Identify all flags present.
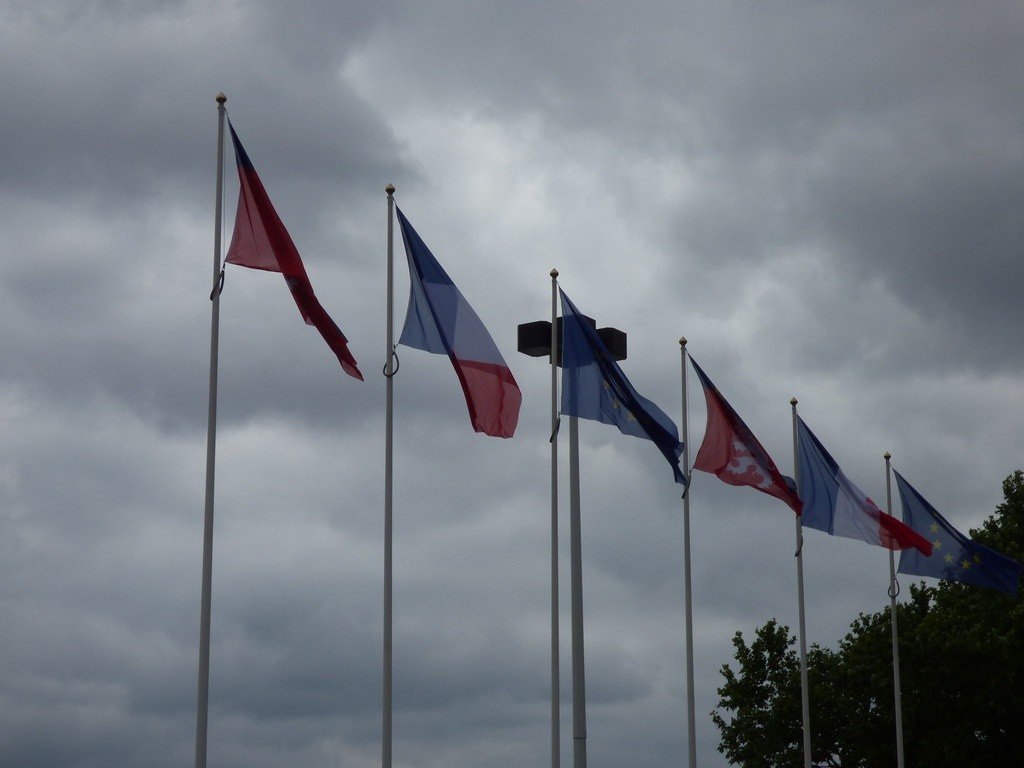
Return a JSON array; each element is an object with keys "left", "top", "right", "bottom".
[
  {"left": 688, "top": 354, "right": 805, "bottom": 517},
  {"left": 225, "top": 118, "right": 364, "bottom": 380},
  {"left": 893, "top": 469, "right": 1024, "bottom": 596},
  {"left": 559, "top": 287, "right": 688, "bottom": 487},
  {"left": 396, "top": 205, "right": 522, "bottom": 438},
  {"left": 797, "top": 415, "right": 933, "bottom": 557}
]
